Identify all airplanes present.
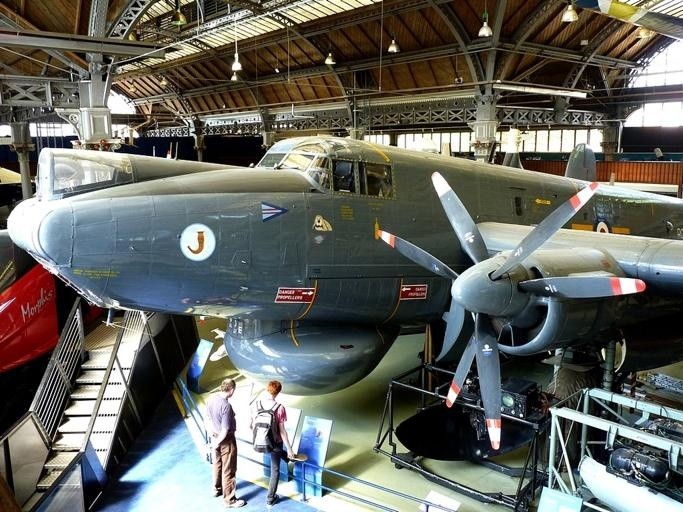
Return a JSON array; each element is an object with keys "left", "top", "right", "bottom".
[{"left": 7, "top": 136, "right": 683, "bottom": 450}]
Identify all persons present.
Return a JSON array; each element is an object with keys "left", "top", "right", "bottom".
[
  {"left": 249, "top": 381, "right": 295, "bottom": 505},
  {"left": 207, "top": 378, "right": 245, "bottom": 508}
]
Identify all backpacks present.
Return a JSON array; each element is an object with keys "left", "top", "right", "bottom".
[{"left": 251, "top": 400, "right": 281, "bottom": 453}]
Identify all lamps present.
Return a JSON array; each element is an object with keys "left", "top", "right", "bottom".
[
  {"left": 478, "top": 0, "right": 492, "bottom": 37},
  {"left": 170, "top": 1, "right": 187, "bottom": 26},
  {"left": 229, "top": 21, "right": 243, "bottom": 81},
  {"left": 636, "top": 27, "right": 652, "bottom": 39},
  {"left": 324, "top": 32, "right": 336, "bottom": 65},
  {"left": 560, "top": 0, "right": 579, "bottom": 23},
  {"left": 387, "top": 16, "right": 399, "bottom": 53}
]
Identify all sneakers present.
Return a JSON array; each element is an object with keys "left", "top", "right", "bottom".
[
  {"left": 265, "top": 494, "right": 280, "bottom": 508},
  {"left": 213, "top": 490, "right": 222, "bottom": 497},
  {"left": 225, "top": 500, "right": 246, "bottom": 509},
  {"left": 287, "top": 454, "right": 308, "bottom": 462}
]
